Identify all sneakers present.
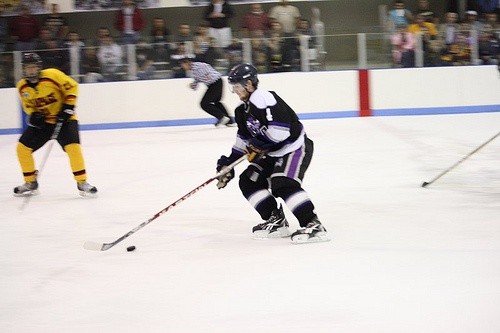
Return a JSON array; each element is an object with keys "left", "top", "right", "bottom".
[
  {"left": 215, "top": 116, "right": 230, "bottom": 128},
  {"left": 292, "top": 214, "right": 331, "bottom": 243},
  {"left": 14, "top": 181, "right": 38, "bottom": 197},
  {"left": 77, "top": 180, "right": 97, "bottom": 197},
  {"left": 226, "top": 119, "right": 238, "bottom": 127},
  {"left": 252, "top": 203, "right": 292, "bottom": 238}
]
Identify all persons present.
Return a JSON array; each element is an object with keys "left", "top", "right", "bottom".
[
  {"left": 181, "top": 56, "right": 235, "bottom": 127},
  {"left": 150, "top": 18, "right": 221, "bottom": 78},
  {"left": 43, "top": 3, "right": 68, "bottom": 43},
  {"left": 12, "top": 52, "right": 99, "bottom": 197},
  {"left": 216, "top": 62, "right": 331, "bottom": 245},
  {"left": 386, "top": 0, "right": 500, "bottom": 67},
  {"left": 202, "top": 0, "right": 325, "bottom": 73},
  {"left": 7, "top": 4, "right": 40, "bottom": 51},
  {"left": 81, "top": 27, "right": 158, "bottom": 83},
  {"left": 112, "top": 0, "right": 145, "bottom": 42},
  {"left": 34, "top": 29, "right": 86, "bottom": 75}
]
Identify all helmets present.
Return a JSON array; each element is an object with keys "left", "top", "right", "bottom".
[
  {"left": 19, "top": 53, "right": 43, "bottom": 80},
  {"left": 179, "top": 57, "right": 193, "bottom": 63},
  {"left": 228, "top": 63, "right": 258, "bottom": 83}
]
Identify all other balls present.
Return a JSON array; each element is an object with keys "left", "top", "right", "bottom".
[{"left": 126, "top": 245, "right": 136, "bottom": 251}]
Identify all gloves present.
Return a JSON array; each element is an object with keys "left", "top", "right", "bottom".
[
  {"left": 56, "top": 104, "right": 74, "bottom": 122},
  {"left": 246, "top": 134, "right": 276, "bottom": 163},
  {"left": 217, "top": 156, "right": 234, "bottom": 189},
  {"left": 30, "top": 112, "right": 46, "bottom": 126}
]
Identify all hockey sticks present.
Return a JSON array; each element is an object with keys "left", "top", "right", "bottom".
[
  {"left": 421, "top": 132, "right": 500, "bottom": 188},
  {"left": 84, "top": 154, "right": 249, "bottom": 251},
  {"left": 15, "top": 117, "right": 64, "bottom": 213}
]
